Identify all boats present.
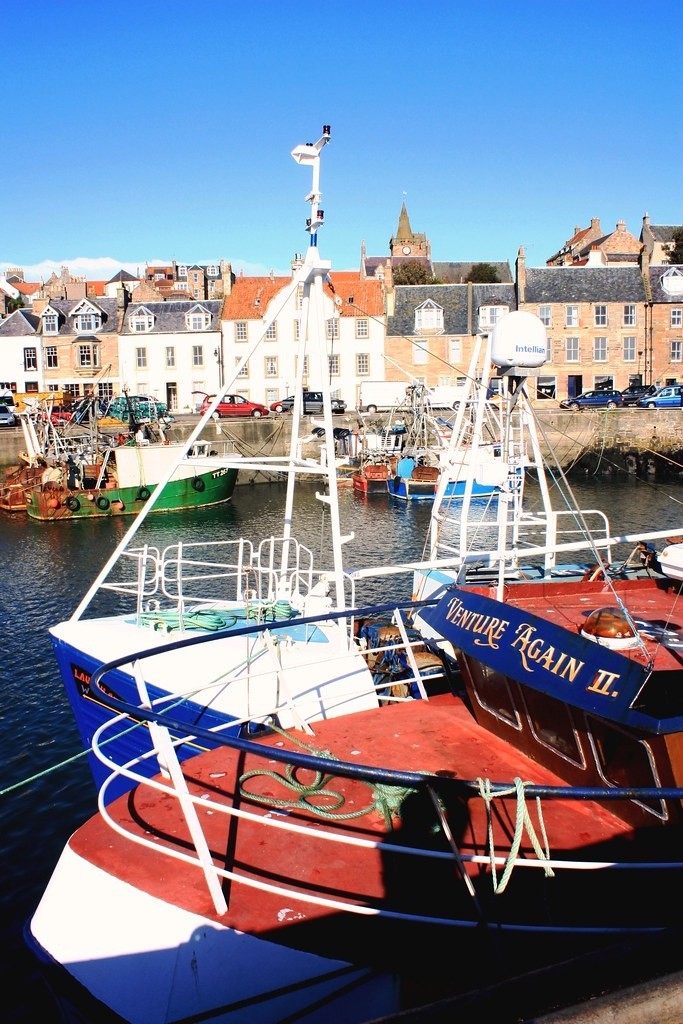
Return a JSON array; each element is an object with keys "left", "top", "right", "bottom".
[
  {"left": 20, "top": 126, "right": 683, "bottom": 1024},
  {"left": 352, "top": 352, "right": 529, "bottom": 500},
  {"left": 0, "top": 363, "right": 243, "bottom": 521}
]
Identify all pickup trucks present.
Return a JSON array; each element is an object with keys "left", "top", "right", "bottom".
[{"left": 282, "top": 391, "right": 347, "bottom": 415}]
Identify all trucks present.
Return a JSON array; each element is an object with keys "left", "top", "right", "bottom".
[{"left": 359, "top": 381, "right": 471, "bottom": 414}]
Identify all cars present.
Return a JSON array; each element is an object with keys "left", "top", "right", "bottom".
[
  {"left": 559, "top": 390, "right": 624, "bottom": 411},
  {"left": 191, "top": 391, "right": 270, "bottom": 419},
  {"left": 0, "top": 404, "right": 15, "bottom": 427},
  {"left": 621, "top": 385, "right": 683, "bottom": 407},
  {"left": 270, "top": 395, "right": 296, "bottom": 413},
  {"left": 42, "top": 406, "right": 72, "bottom": 426}
]
